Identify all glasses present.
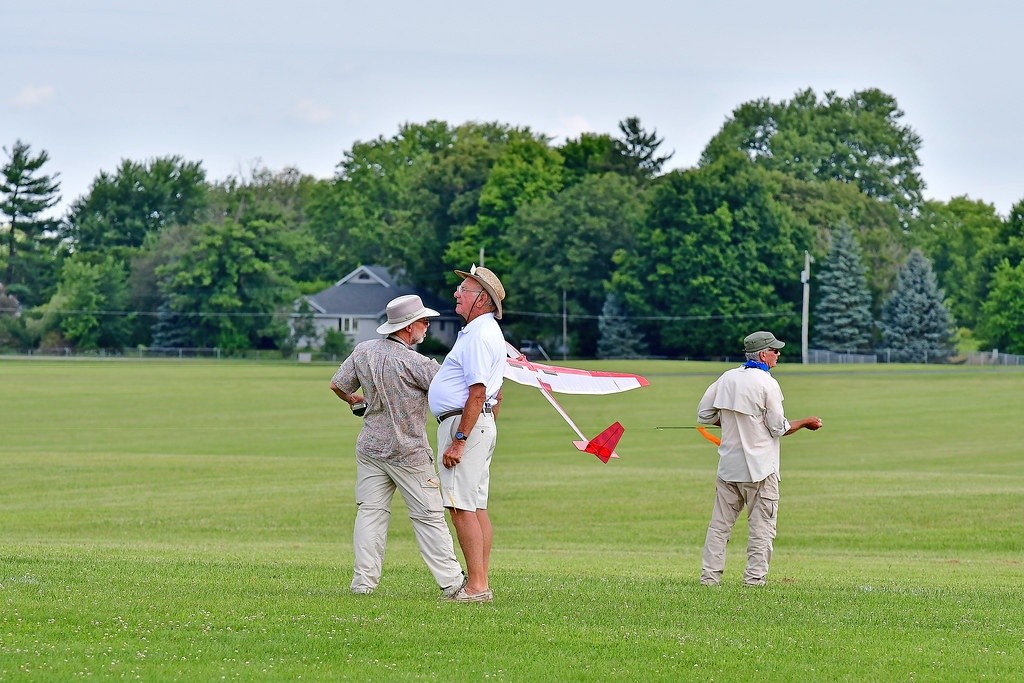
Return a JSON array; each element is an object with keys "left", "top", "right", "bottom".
[
  {"left": 769, "top": 348, "right": 779, "bottom": 354},
  {"left": 457, "top": 285, "right": 488, "bottom": 296},
  {"left": 415, "top": 318, "right": 429, "bottom": 326}
]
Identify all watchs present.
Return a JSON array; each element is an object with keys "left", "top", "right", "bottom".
[{"left": 455, "top": 431, "right": 467, "bottom": 441}]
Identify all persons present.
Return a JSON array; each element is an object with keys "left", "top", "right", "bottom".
[
  {"left": 697, "top": 331, "right": 822, "bottom": 586},
  {"left": 330, "top": 295, "right": 469, "bottom": 597},
  {"left": 428, "top": 267, "right": 506, "bottom": 601}
]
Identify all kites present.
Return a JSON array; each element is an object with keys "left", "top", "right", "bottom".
[{"left": 500, "top": 340, "right": 649, "bottom": 464}]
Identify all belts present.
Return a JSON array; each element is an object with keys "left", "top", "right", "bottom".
[{"left": 437, "top": 407, "right": 491, "bottom": 424}]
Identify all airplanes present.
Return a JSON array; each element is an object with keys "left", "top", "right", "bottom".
[{"left": 502, "top": 341, "right": 650, "bottom": 465}]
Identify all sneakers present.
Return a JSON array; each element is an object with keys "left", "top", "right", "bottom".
[{"left": 444, "top": 588, "right": 494, "bottom": 602}]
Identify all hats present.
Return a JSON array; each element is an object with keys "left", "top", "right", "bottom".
[
  {"left": 744, "top": 332, "right": 785, "bottom": 353},
  {"left": 454, "top": 263, "right": 505, "bottom": 320},
  {"left": 376, "top": 295, "right": 441, "bottom": 335}
]
